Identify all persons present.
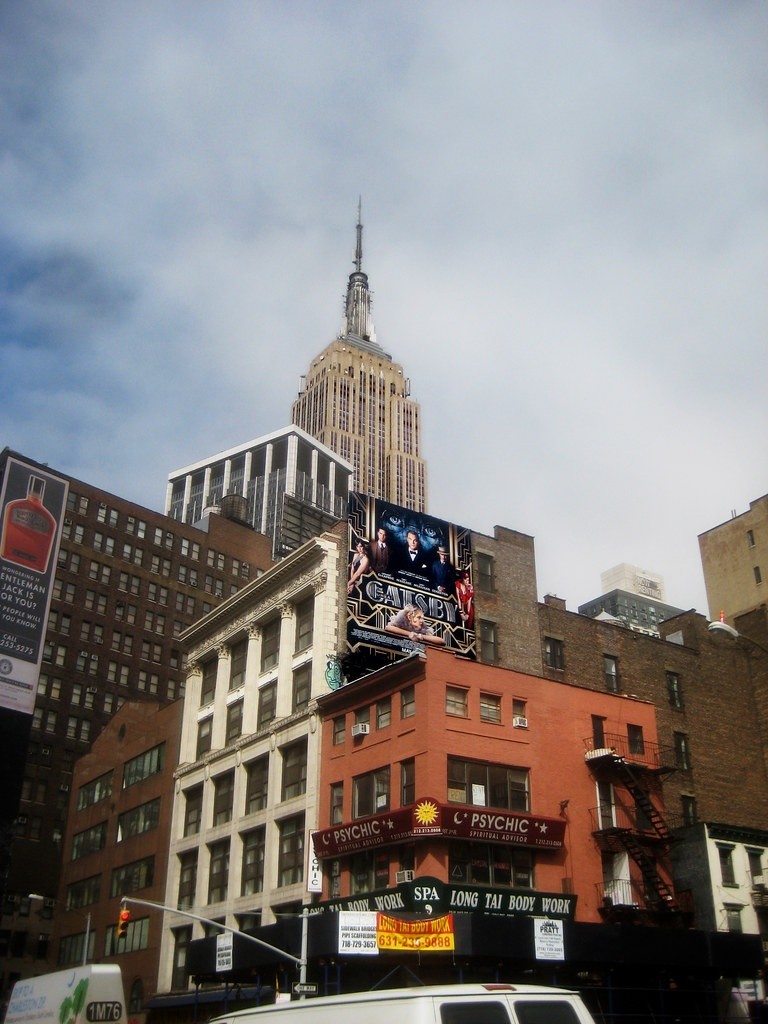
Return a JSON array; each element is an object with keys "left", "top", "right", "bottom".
[
  {"left": 454, "top": 573, "right": 474, "bottom": 629},
  {"left": 430, "top": 545, "right": 456, "bottom": 597},
  {"left": 347, "top": 538, "right": 370, "bottom": 598},
  {"left": 368, "top": 526, "right": 390, "bottom": 575},
  {"left": 385, "top": 602, "right": 446, "bottom": 644},
  {"left": 390, "top": 530, "right": 447, "bottom": 592}
]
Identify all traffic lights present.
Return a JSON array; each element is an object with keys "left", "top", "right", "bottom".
[{"left": 117, "top": 909, "right": 129, "bottom": 938}]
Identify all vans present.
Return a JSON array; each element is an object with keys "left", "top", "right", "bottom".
[{"left": 211, "top": 984, "right": 596, "bottom": 1024}]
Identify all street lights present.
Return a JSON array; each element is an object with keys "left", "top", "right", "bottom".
[{"left": 30, "top": 894, "right": 92, "bottom": 967}]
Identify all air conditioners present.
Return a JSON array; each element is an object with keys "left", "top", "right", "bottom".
[
  {"left": 395, "top": 871, "right": 413, "bottom": 883},
  {"left": 513, "top": 717, "right": 527, "bottom": 728},
  {"left": 351, "top": 724, "right": 369, "bottom": 736},
  {"left": 10, "top": 500, "right": 250, "bottom": 909}
]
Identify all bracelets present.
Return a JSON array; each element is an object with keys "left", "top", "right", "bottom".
[
  {"left": 459, "top": 608, "right": 463, "bottom": 615},
  {"left": 409, "top": 632, "right": 417, "bottom": 639},
  {"left": 418, "top": 633, "right": 424, "bottom": 640}
]
